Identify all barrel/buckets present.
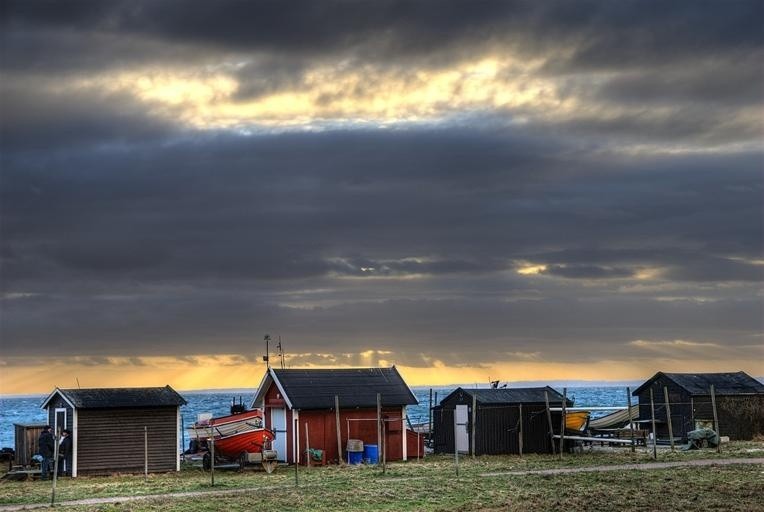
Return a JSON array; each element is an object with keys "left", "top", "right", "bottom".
[
  {"left": 364, "top": 443, "right": 380, "bottom": 465},
  {"left": 346, "top": 450, "right": 364, "bottom": 465}
]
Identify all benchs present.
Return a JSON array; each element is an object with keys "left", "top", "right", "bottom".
[{"left": 611, "top": 425, "right": 649, "bottom": 447}]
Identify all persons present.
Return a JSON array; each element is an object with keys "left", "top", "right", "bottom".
[
  {"left": 59, "top": 430, "right": 72, "bottom": 464},
  {"left": 38, "top": 425, "right": 54, "bottom": 481}
]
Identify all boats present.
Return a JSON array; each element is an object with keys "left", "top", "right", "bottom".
[
  {"left": 565, "top": 404, "right": 639, "bottom": 431},
  {"left": 188, "top": 409, "right": 275, "bottom": 459}
]
